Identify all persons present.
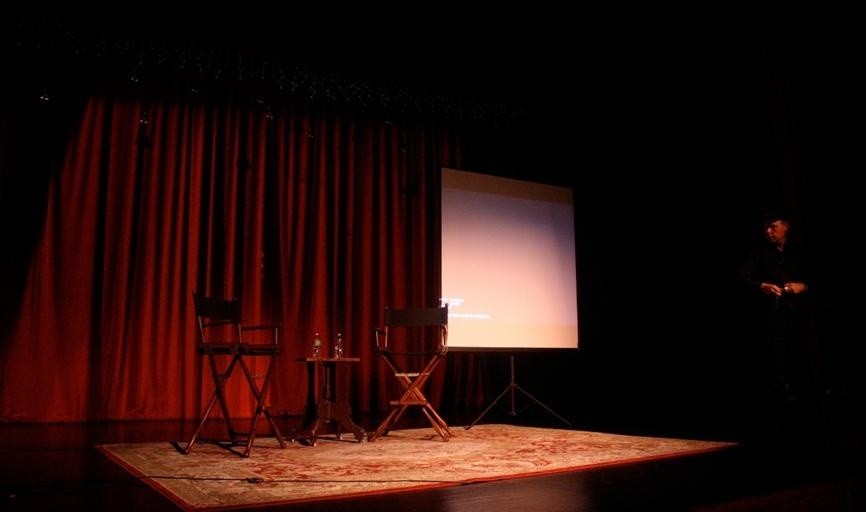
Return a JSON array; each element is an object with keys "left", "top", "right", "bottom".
[{"left": 737, "top": 209, "right": 815, "bottom": 304}]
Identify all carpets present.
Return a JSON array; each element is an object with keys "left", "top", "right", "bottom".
[{"left": 97, "top": 422, "right": 739, "bottom": 512}]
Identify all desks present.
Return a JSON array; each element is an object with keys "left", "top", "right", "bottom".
[{"left": 286, "top": 358, "right": 368, "bottom": 446}]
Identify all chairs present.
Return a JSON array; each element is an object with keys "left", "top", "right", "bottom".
[
  {"left": 185, "top": 289, "right": 287, "bottom": 457},
  {"left": 368, "top": 302, "right": 457, "bottom": 442}
]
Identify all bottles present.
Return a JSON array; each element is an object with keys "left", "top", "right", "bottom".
[
  {"left": 334, "top": 332, "right": 344, "bottom": 360},
  {"left": 311, "top": 332, "right": 323, "bottom": 358}
]
